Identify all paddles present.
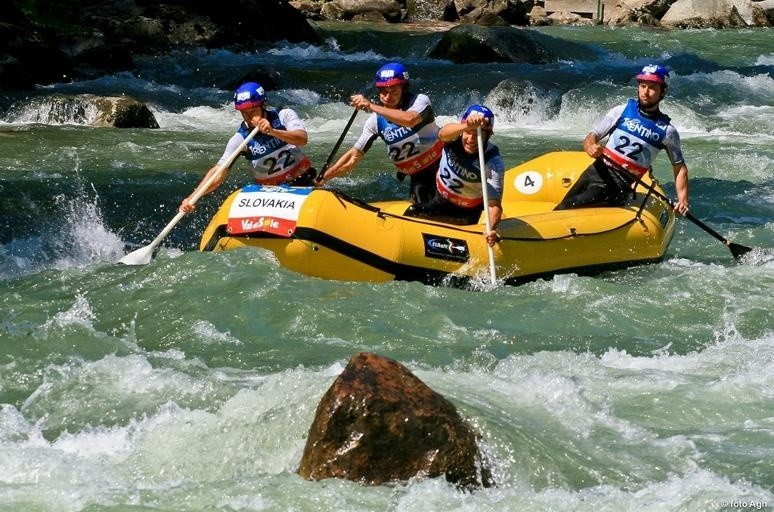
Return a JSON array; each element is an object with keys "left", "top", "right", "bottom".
[
  {"left": 117, "top": 124, "right": 261, "bottom": 266},
  {"left": 599, "top": 155, "right": 753, "bottom": 261}
]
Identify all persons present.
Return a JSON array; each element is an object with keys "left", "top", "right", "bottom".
[
  {"left": 311, "top": 62, "right": 443, "bottom": 203},
  {"left": 552, "top": 64, "right": 691, "bottom": 217},
  {"left": 175, "top": 82, "right": 318, "bottom": 214},
  {"left": 404, "top": 104, "right": 504, "bottom": 245}
]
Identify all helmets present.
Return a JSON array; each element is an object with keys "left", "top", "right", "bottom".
[
  {"left": 461, "top": 105, "right": 495, "bottom": 132},
  {"left": 636, "top": 64, "right": 670, "bottom": 89},
  {"left": 235, "top": 81, "right": 265, "bottom": 110},
  {"left": 374, "top": 63, "right": 410, "bottom": 88}
]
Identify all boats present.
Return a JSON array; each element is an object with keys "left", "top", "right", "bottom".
[{"left": 197, "top": 150, "right": 680, "bottom": 289}]
